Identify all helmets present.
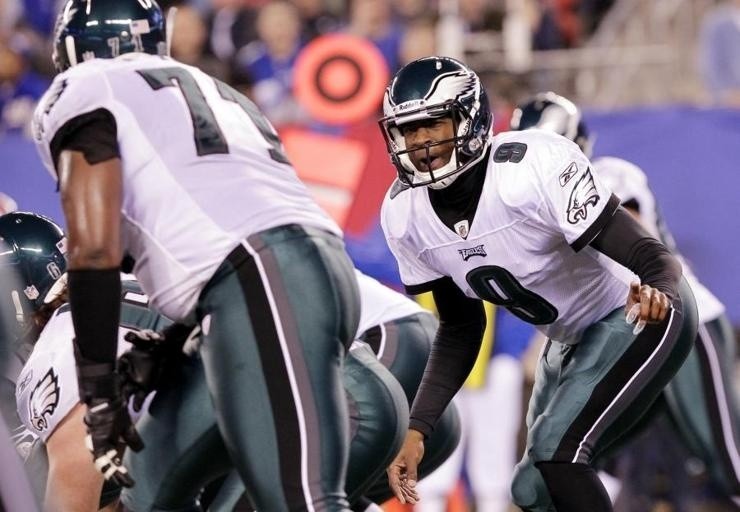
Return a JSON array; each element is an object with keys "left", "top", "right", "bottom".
[
  {"left": 0, "top": 211, "right": 65, "bottom": 323},
  {"left": 51, "top": 1, "right": 166, "bottom": 72},
  {"left": 509, "top": 90, "right": 590, "bottom": 141},
  {"left": 377, "top": 56, "right": 493, "bottom": 174}
]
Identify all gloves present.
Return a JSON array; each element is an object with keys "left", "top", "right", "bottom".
[{"left": 73, "top": 330, "right": 188, "bottom": 489}]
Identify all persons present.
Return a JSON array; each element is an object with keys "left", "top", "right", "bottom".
[
  {"left": 511, "top": 91, "right": 738, "bottom": 512},
  {"left": 29, "top": 1, "right": 359, "bottom": 510},
  {"left": 380, "top": 58, "right": 699, "bottom": 511},
  {"left": 2, "top": 211, "right": 534, "bottom": 511},
  {"left": 1, "top": 2, "right": 617, "bottom": 133}
]
